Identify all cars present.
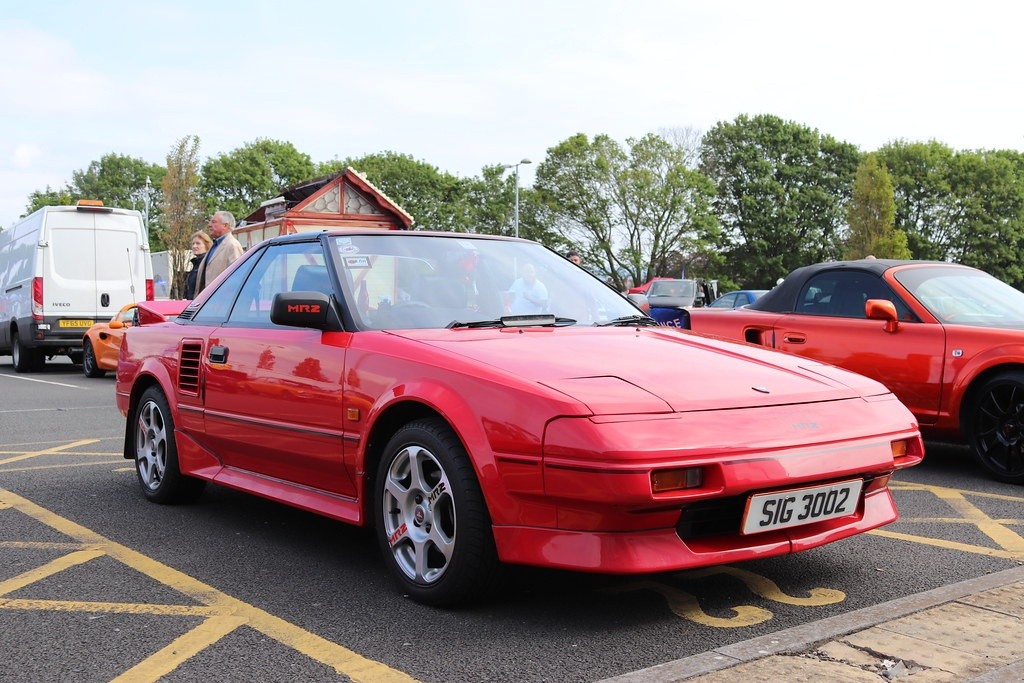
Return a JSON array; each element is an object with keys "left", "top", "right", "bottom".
[
  {"left": 708, "top": 289, "right": 819, "bottom": 309},
  {"left": 646, "top": 278, "right": 713, "bottom": 312}
]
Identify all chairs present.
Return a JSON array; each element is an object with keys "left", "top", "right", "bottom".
[
  {"left": 289, "top": 264, "right": 336, "bottom": 301},
  {"left": 829, "top": 286, "right": 854, "bottom": 316}
]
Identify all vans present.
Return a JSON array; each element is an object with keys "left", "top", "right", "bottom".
[
  {"left": 1, "top": 200, "right": 155, "bottom": 373},
  {"left": 150, "top": 251, "right": 195, "bottom": 299}
]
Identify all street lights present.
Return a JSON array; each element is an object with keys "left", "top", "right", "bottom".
[{"left": 502, "top": 158, "right": 533, "bottom": 276}]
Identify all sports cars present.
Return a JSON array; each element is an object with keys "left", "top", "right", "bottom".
[
  {"left": 680, "top": 259, "right": 1024, "bottom": 487},
  {"left": 113, "top": 222, "right": 925, "bottom": 604},
  {"left": 81, "top": 305, "right": 142, "bottom": 379}
]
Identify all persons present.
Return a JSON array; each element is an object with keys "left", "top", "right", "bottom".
[
  {"left": 194, "top": 211, "right": 244, "bottom": 301},
  {"left": 184, "top": 230, "right": 214, "bottom": 301},
  {"left": 566, "top": 252, "right": 581, "bottom": 267},
  {"left": 504, "top": 263, "right": 549, "bottom": 315}
]
